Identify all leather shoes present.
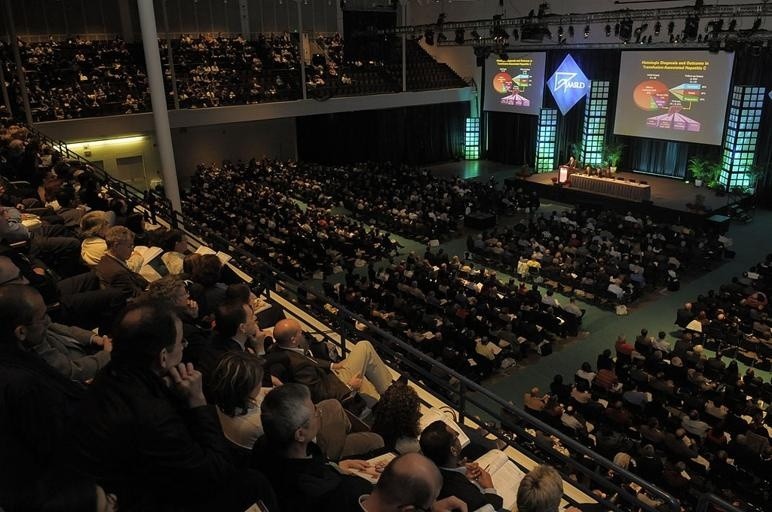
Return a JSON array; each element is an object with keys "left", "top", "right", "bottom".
[{"left": 393, "top": 371, "right": 411, "bottom": 388}]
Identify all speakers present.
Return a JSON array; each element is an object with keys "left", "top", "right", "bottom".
[
  {"left": 706, "top": 214, "right": 730, "bottom": 233},
  {"left": 504, "top": 176, "right": 515, "bottom": 186}
]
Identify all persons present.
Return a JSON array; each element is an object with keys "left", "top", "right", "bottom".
[{"left": 0, "top": 32, "right": 772, "bottom": 512}]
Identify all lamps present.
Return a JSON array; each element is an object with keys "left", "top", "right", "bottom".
[{"left": 408, "top": 1, "right": 772, "bottom": 58}]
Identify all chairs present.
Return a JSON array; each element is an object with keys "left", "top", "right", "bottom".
[
  {"left": 0, "top": 31, "right": 467, "bottom": 124},
  {"left": 0, "top": 126, "right": 772, "bottom": 512}
]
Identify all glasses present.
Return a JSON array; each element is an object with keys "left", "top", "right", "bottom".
[
  {"left": 126, "top": 244, "right": 136, "bottom": 250},
  {"left": 298, "top": 404, "right": 322, "bottom": 428},
  {"left": 449, "top": 431, "right": 461, "bottom": 446},
  {"left": 169, "top": 338, "right": 189, "bottom": 354}
]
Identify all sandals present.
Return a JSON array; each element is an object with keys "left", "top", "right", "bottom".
[
  {"left": 477, "top": 420, "right": 496, "bottom": 438},
  {"left": 496, "top": 437, "right": 509, "bottom": 451}
]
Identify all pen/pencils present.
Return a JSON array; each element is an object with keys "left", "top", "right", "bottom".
[{"left": 476, "top": 464, "right": 489, "bottom": 481}]
